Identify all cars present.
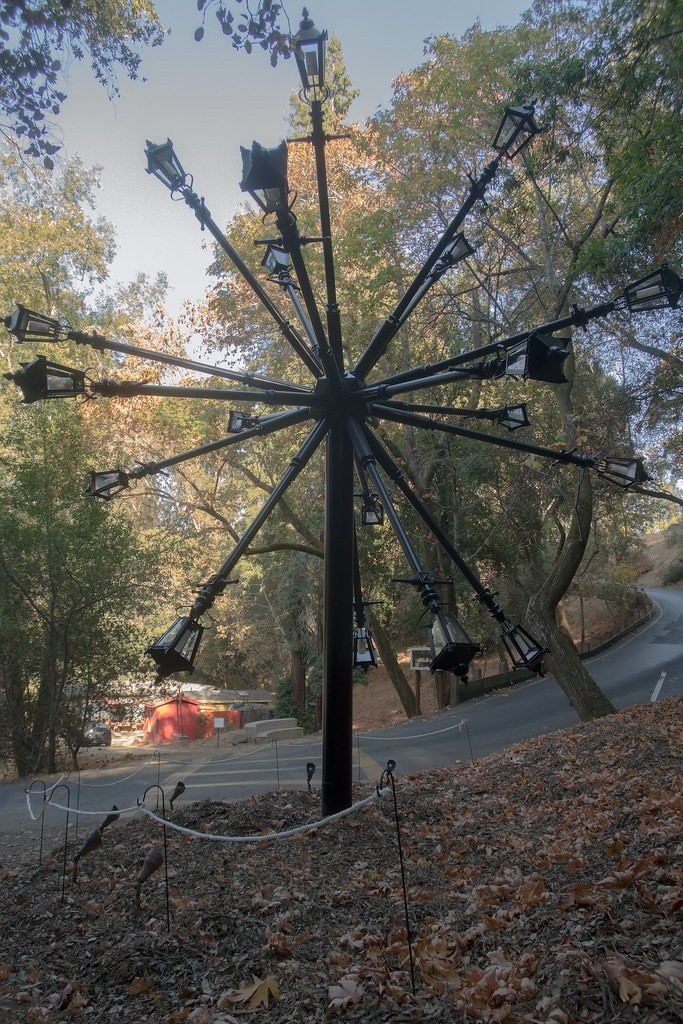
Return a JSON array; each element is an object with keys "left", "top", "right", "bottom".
[{"left": 70, "top": 722, "right": 111, "bottom": 748}]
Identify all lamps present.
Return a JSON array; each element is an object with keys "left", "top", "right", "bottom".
[
  {"left": 73, "top": 828, "right": 101, "bottom": 882},
  {"left": 100, "top": 805, "right": 121, "bottom": 830},
  {"left": 386, "top": 759, "right": 396, "bottom": 784},
  {"left": 306, "top": 763, "right": 316, "bottom": 791},
  {"left": 133, "top": 847, "right": 164, "bottom": 915},
  {"left": 169, "top": 781, "right": 185, "bottom": 810}
]
